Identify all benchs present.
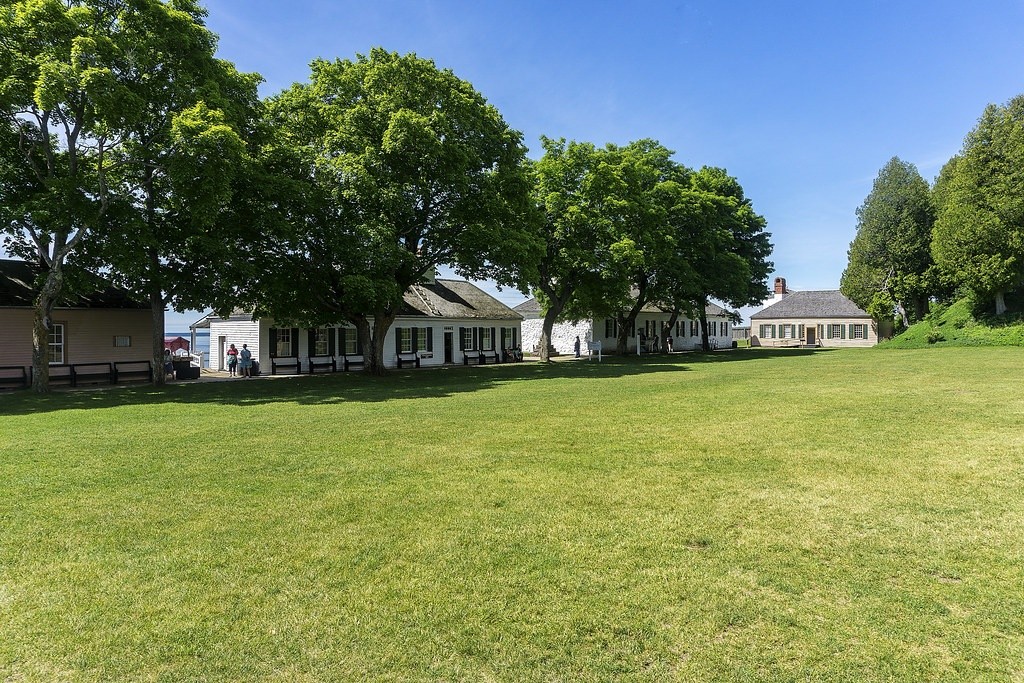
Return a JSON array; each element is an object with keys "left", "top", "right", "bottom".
[
  {"left": 309, "top": 356, "right": 337, "bottom": 374},
  {"left": 272, "top": 356, "right": 301, "bottom": 375},
  {"left": 72, "top": 362, "right": 113, "bottom": 385},
  {"left": 463, "top": 349, "right": 481, "bottom": 365},
  {"left": 30, "top": 364, "right": 75, "bottom": 386},
  {"left": 0, "top": 366, "right": 28, "bottom": 389},
  {"left": 481, "top": 350, "right": 500, "bottom": 364},
  {"left": 396, "top": 352, "right": 421, "bottom": 368},
  {"left": 114, "top": 361, "right": 153, "bottom": 383},
  {"left": 502, "top": 349, "right": 520, "bottom": 363},
  {"left": 344, "top": 355, "right": 365, "bottom": 371}
]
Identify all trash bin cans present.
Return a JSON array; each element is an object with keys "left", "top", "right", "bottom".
[
  {"left": 237, "top": 358, "right": 256, "bottom": 376},
  {"left": 732, "top": 341, "right": 737, "bottom": 348}
]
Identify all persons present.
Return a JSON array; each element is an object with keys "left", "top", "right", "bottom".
[
  {"left": 240, "top": 344, "right": 252, "bottom": 378},
  {"left": 507, "top": 346, "right": 517, "bottom": 363},
  {"left": 668, "top": 336, "right": 673, "bottom": 352},
  {"left": 227, "top": 344, "right": 238, "bottom": 377},
  {"left": 574, "top": 336, "right": 580, "bottom": 358},
  {"left": 164, "top": 350, "right": 174, "bottom": 381},
  {"left": 653, "top": 335, "right": 659, "bottom": 352},
  {"left": 515, "top": 344, "right": 524, "bottom": 362}
]
